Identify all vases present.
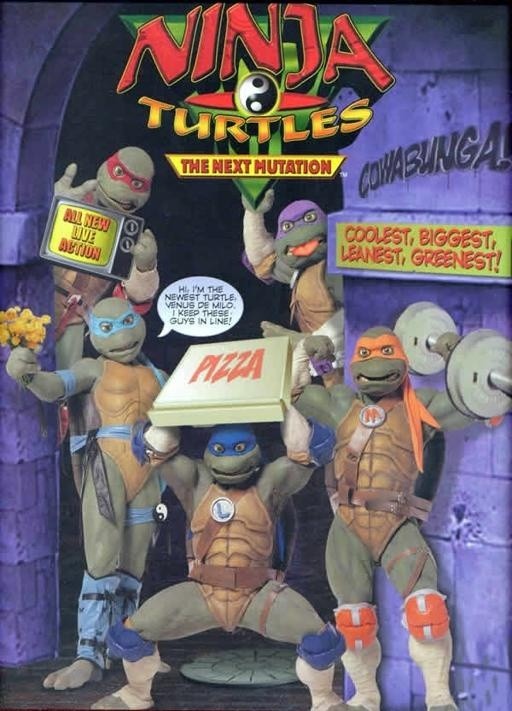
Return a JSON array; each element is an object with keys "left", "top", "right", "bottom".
[{"left": 0, "top": 304, "right": 52, "bottom": 389}]
